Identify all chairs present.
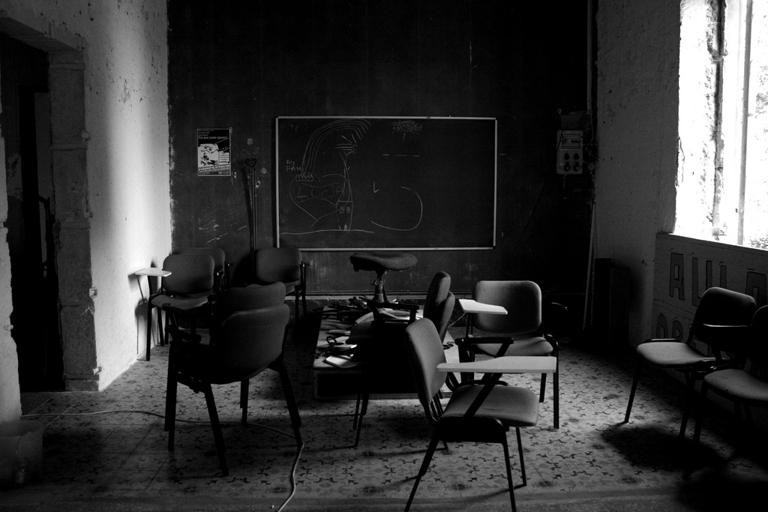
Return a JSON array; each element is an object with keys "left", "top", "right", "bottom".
[
  {"left": 624, "top": 287, "right": 757, "bottom": 438},
  {"left": 162, "top": 283, "right": 286, "bottom": 431},
  {"left": 679, "top": 305, "right": 766, "bottom": 481},
  {"left": 168, "top": 305, "right": 304, "bottom": 477},
  {"left": 472, "top": 279, "right": 561, "bottom": 433},
  {"left": 179, "top": 250, "right": 232, "bottom": 293},
  {"left": 351, "top": 272, "right": 458, "bottom": 455},
  {"left": 146, "top": 253, "right": 215, "bottom": 361},
  {"left": 255, "top": 248, "right": 308, "bottom": 324},
  {"left": 404, "top": 317, "right": 537, "bottom": 511}
]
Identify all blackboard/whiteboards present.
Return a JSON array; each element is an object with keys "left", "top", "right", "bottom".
[{"left": 276, "top": 116, "right": 497, "bottom": 251}]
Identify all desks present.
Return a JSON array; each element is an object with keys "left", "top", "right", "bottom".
[{"left": 350, "top": 252, "right": 418, "bottom": 303}]
